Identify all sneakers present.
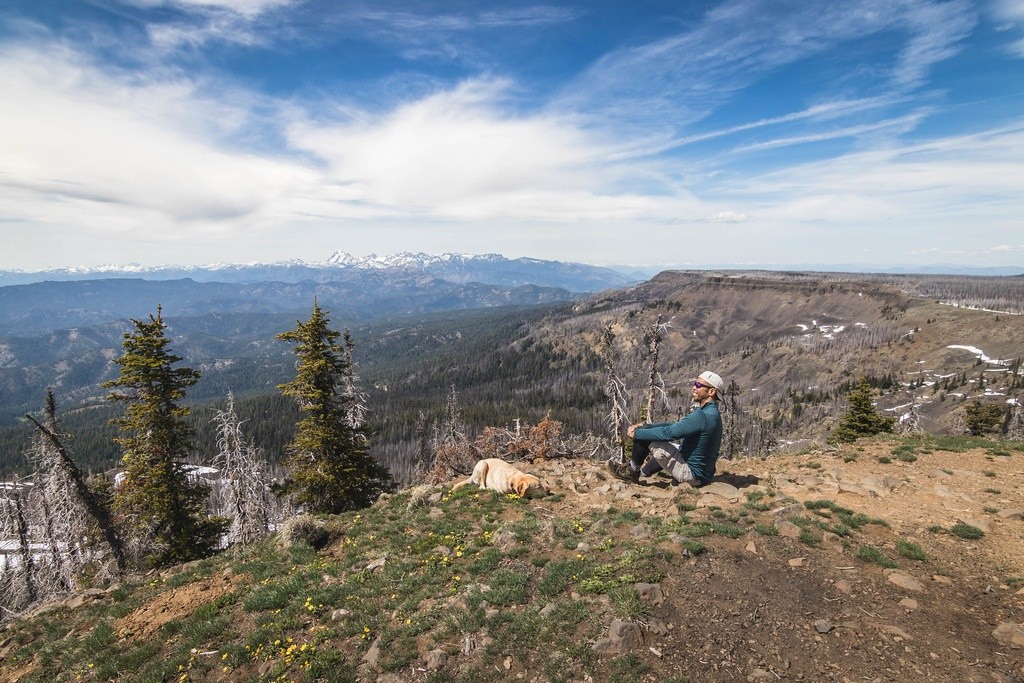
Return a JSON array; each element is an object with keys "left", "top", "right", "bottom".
[
  {"left": 640, "top": 455, "right": 650, "bottom": 477},
  {"left": 605, "top": 460, "right": 640, "bottom": 484}
]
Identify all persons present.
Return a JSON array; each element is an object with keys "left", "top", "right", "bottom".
[{"left": 606, "top": 371, "right": 725, "bottom": 487}]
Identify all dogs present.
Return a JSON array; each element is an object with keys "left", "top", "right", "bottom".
[{"left": 451, "top": 458, "right": 550, "bottom": 502}]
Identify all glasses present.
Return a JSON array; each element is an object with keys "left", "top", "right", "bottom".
[{"left": 694, "top": 381, "right": 715, "bottom": 389}]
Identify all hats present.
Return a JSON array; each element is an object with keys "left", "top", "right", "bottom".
[{"left": 698, "top": 371, "right": 724, "bottom": 402}]
